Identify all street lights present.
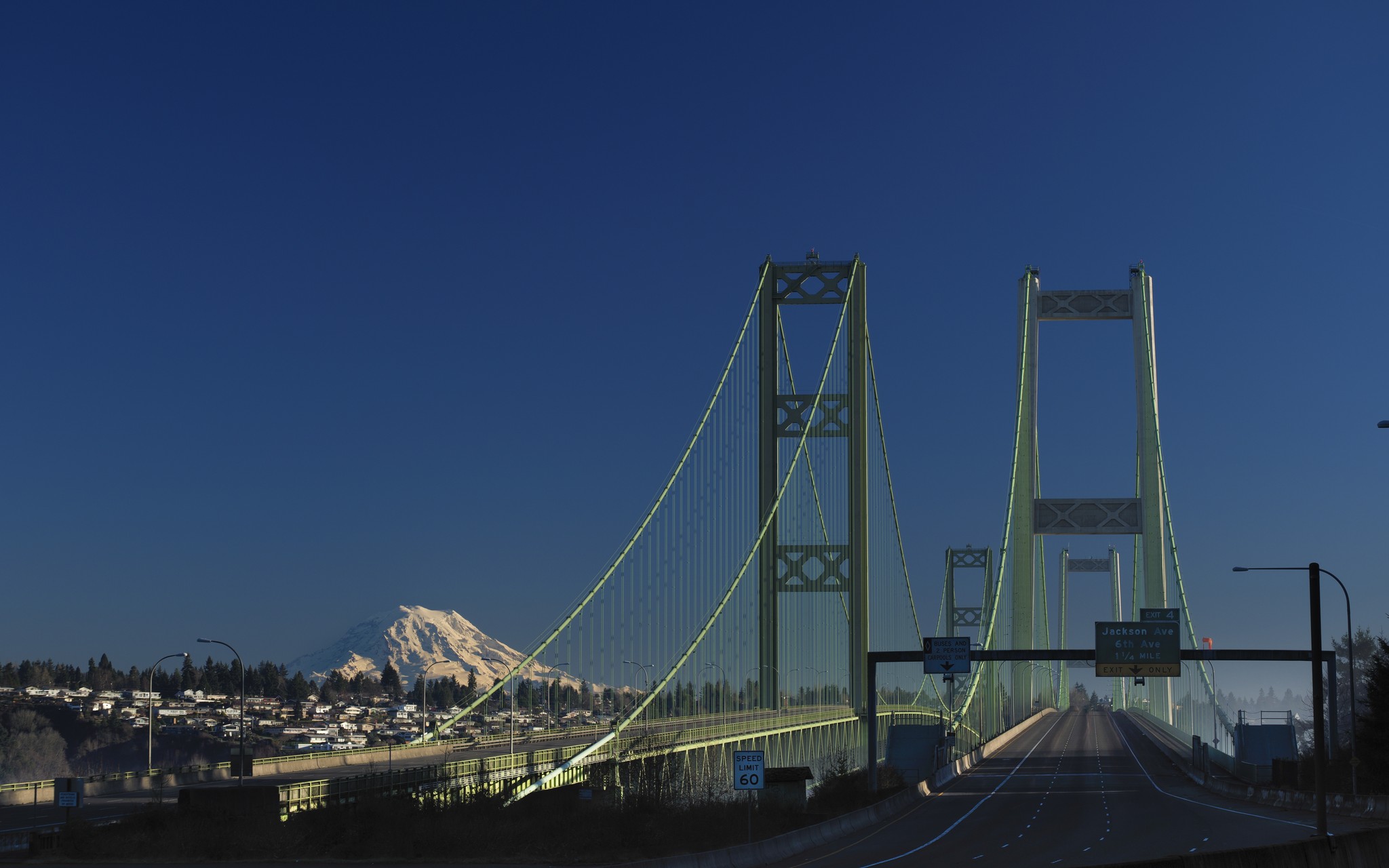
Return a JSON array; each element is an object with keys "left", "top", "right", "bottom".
[
  {"left": 887, "top": 673, "right": 899, "bottom": 705},
  {"left": 905, "top": 676, "right": 916, "bottom": 709},
  {"left": 548, "top": 663, "right": 571, "bottom": 730},
  {"left": 746, "top": 668, "right": 760, "bottom": 710},
  {"left": 623, "top": 661, "right": 654, "bottom": 735},
  {"left": 876, "top": 673, "right": 888, "bottom": 706},
  {"left": 953, "top": 697, "right": 960, "bottom": 709},
  {"left": 149, "top": 653, "right": 188, "bottom": 778},
  {"left": 1105, "top": 680, "right": 1129, "bottom": 711},
  {"left": 838, "top": 668, "right": 851, "bottom": 706},
  {"left": 920, "top": 681, "right": 929, "bottom": 708},
  {"left": 197, "top": 638, "right": 246, "bottom": 786},
  {"left": 481, "top": 657, "right": 513, "bottom": 753},
  {"left": 805, "top": 667, "right": 829, "bottom": 713},
  {"left": 422, "top": 660, "right": 451, "bottom": 741},
  {"left": 763, "top": 666, "right": 780, "bottom": 718},
  {"left": 698, "top": 664, "right": 726, "bottom": 724},
  {"left": 787, "top": 669, "right": 799, "bottom": 711},
  {"left": 1012, "top": 661, "right": 1070, "bottom": 726},
  {"left": 949, "top": 643, "right": 983, "bottom": 762},
  {"left": 934, "top": 686, "right": 941, "bottom": 708},
  {"left": 1233, "top": 566, "right": 1358, "bottom": 794}
]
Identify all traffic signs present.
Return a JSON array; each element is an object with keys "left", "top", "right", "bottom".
[
  {"left": 922, "top": 637, "right": 971, "bottom": 675},
  {"left": 1095, "top": 621, "right": 1182, "bottom": 677}
]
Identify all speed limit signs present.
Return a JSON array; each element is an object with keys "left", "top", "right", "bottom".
[{"left": 734, "top": 750, "right": 766, "bottom": 790}]
